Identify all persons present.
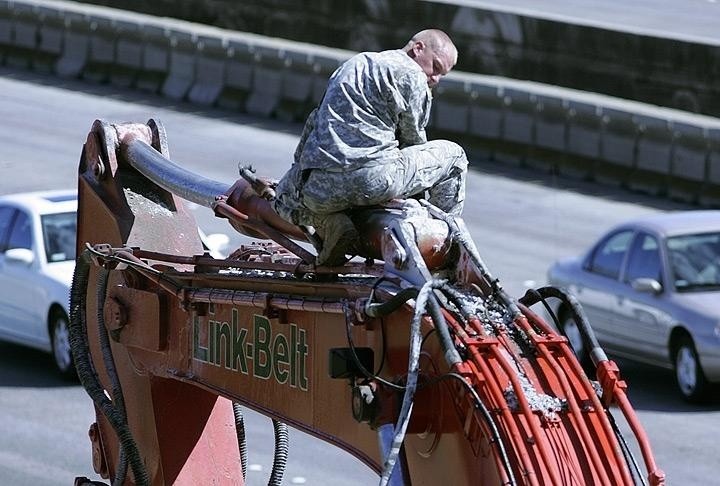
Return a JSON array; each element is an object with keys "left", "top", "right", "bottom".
[{"left": 273, "top": 25, "right": 469, "bottom": 283}]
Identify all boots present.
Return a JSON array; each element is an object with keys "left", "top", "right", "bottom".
[{"left": 310, "top": 211, "right": 359, "bottom": 267}]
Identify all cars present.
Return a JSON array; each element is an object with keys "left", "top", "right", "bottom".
[
  {"left": 542, "top": 208, "right": 719, "bottom": 406},
  {"left": 0, "top": 189, "right": 231, "bottom": 376}
]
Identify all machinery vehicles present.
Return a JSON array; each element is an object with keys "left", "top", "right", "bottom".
[{"left": 70, "top": 118, "right": 665, "bottom": 486}]
